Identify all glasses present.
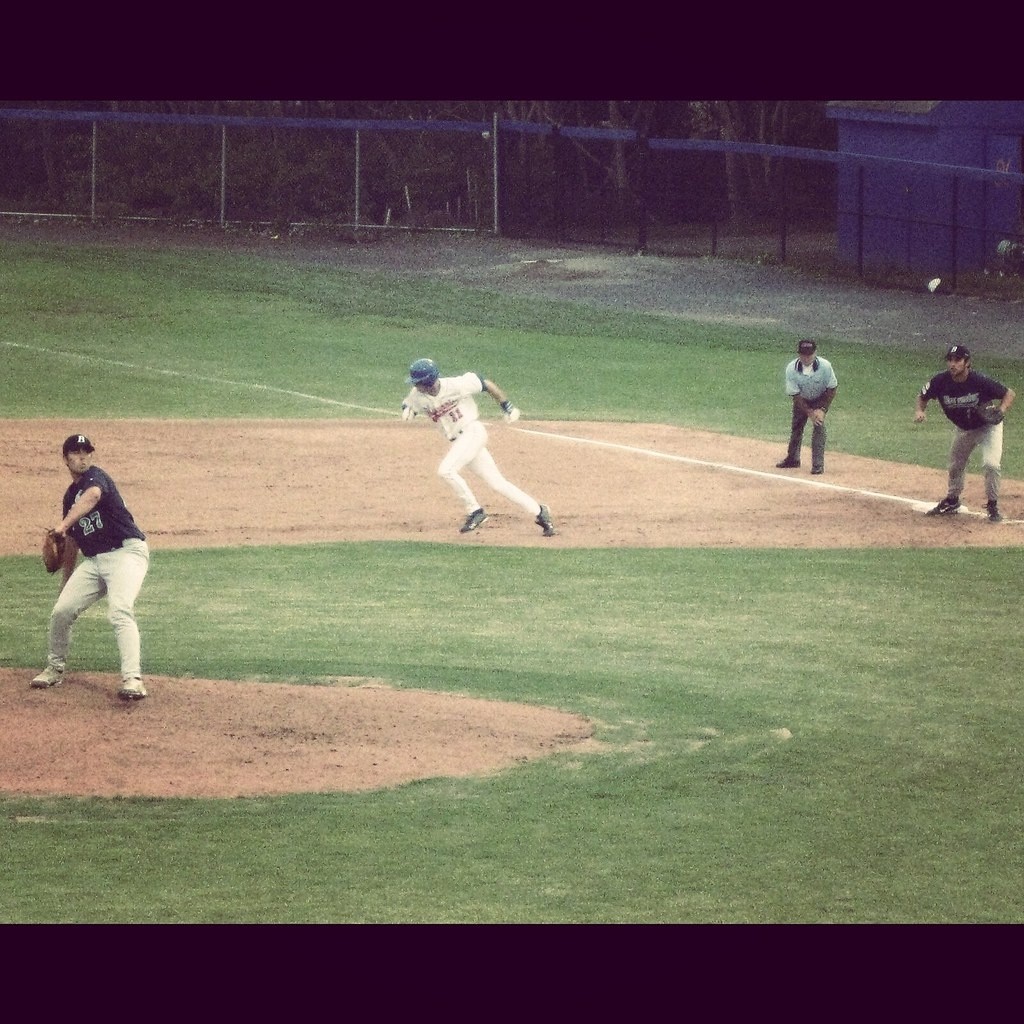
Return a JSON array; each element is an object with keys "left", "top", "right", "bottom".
[{"left": 67, "top": 450, "right": 90, "bottom": 456}]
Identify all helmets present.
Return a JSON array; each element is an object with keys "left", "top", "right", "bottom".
[{"left": 405, "top": 358, "right": 440, "bottom": 387}]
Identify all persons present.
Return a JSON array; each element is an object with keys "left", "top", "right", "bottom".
[
  {"left": 28, "top": 434, "right": 150, "bottom": 701},
  {"left": 914, "top": 347, "right": 1016, "bottom": 522},
  {"left": 776, "top": 340, "right": 839, "bottom": 475},
  {"left": 401, "top": 358, "right": 555, "bottom": 537}
]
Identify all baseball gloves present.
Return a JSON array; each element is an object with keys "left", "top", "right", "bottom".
[
  {"left": 977, "top": 400, "right": 1005, "bottom": 426},
  {"left": 42, "top": 525, "right": 66, "bottom": 577}
]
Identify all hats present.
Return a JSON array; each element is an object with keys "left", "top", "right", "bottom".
[
  {"left": 63, "top": 434, "right": 95, "bottom": 456},
  {"left": 945, "top": 345, "right": 970, "bottom": 359},
  {"left": 797, "top": 340, "right": 817, "bottom": 355}
]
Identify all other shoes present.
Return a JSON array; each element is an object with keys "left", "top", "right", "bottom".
[
  {"left": 31, "top": 666, "right": 65, "bottom": 689},
  {"left": 118, "top": 677, "right": 147, "bottom": 699},
  {"left": 534, "top": 505, "right": 555, "bottom": 537},
  {"left": 776, "top": 456, "right": 800, "bottom": 468},
  {"left": 811, "top": 466, "right": 824, "bottom": 474},
  {"left": 982, "top": 500, "right": 1003, "bottom": 522},
  {"left": 460, "top": 508, "right": 488, "bottom": 533},
  {"left": 926, "top": 496, "right": 962, "bottom": 516}
]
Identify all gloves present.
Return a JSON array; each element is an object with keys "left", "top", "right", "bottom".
[
  {"left": 504, "top": 407, "right": 520, "bottom": 424},
  {"left": 401, "top": 407, "right": 415, "bottom": 422}
]
